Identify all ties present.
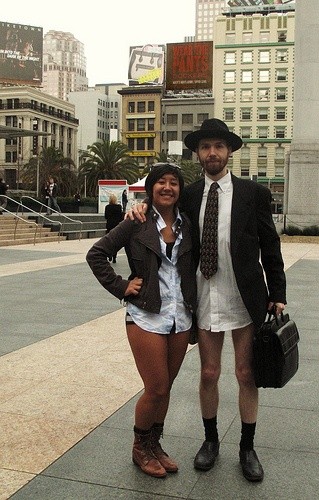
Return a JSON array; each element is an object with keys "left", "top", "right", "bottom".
[{"left": 200, "top": 182, "right": 219, "bottom": 280}]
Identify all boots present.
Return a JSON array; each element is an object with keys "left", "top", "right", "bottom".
[
  {"left": 132, "top": 425, "right": 167, "bottom": 478},
  {"left": 151, "top": 424, "right": 178, "bottom": 473}
]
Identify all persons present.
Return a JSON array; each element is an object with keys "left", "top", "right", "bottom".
[
  {"left": 74, "top": 191, "right": 81, "bottom": 202},
  {"left": 85, "top": 163, "right": 193, "bottom": 478},
  {"left": 122, "top": 190, "right": 128, "bottom": 213},
  {"left": 46, "top": 177, "right": 61, "bottom": 215},
  {"left": 104, "top": 194, "right": 123, "bottom": 264},
  {"left": 124, "top": 117, "right": 286, "bottom": 482},
  {"left": 40, "top": 181, "right": 49, "bottom": 214},
  {"left": 0, "top": 178, "right": 9, "bottom": 214}
]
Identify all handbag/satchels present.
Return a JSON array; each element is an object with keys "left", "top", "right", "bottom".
[{"left": 251, "top": 305, "right": 301, "bottom": 388}]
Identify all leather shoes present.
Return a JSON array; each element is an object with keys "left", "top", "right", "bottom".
[
  {"left": 239, "top": 450, "right": 264, "bottom": 481},
  {"left": 194, "top": 440, "right": 220, "bottom": 469}
]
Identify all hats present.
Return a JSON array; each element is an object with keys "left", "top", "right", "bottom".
[{"left": 185, "top": 119, "right": 243, "bottom": 152}]
